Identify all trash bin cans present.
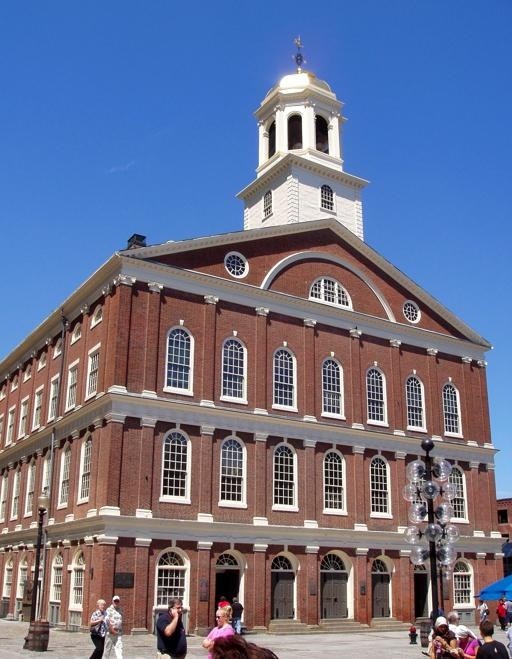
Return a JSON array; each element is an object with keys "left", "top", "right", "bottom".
[
  {"left": 419, "top": 622, "right": 432, "bottom": 647},
  {"left": 28, "top": 621, "right": 49, "bottom": 652}
]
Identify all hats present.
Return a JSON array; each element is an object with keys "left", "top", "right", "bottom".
[
  {"left": 112, "top": 595, "right": 121, "bottom": 602},
  {"left": 435, "top": 615, "right": 449, "bottom": 629}
]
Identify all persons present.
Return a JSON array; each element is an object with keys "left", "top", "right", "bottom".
[
  {"left": 89, "top": 599, "right": 116, "bottom": 659},
  {"left": 101, "top": 595, "right": 123, "bottom": 659},
  {"left": 155, "top": 599, "right": 187, "bottom": 659},
  {"left": 427, "top": 596, "right": 512, "bottom": 659},
  {"left": 202, "top": 596, "right": 280, "bottom": 659}
]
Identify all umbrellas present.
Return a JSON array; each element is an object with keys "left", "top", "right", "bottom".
[{"left": 473, "top": 575, "right": 512, "bottom": 602}]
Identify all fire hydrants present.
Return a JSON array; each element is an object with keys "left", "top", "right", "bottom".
[{"left": 408, "top": 624, "right": 420, "bottom": 645}]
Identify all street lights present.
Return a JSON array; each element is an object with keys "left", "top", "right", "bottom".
[
  {"left": 401, "top": 435, "right": 460, "bottom": 649},
  {"left": 19, "top": 490, "right": 51, "bottom": 649}
]
